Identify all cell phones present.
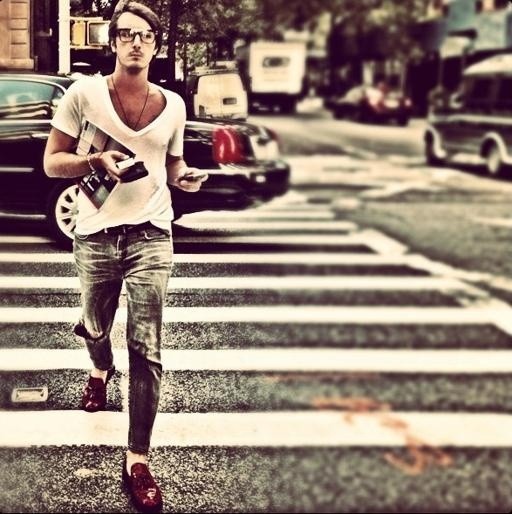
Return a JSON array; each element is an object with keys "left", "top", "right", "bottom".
[{"left": 177, "top": 173, "right": 207, "bottom": 183}]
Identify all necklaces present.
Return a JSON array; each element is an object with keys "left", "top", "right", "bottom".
[{"left": 110, "top": 72, "right": 151, "bottom": 131}]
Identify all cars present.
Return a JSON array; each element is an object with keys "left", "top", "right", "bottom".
[
  {"left": 0, "top": 71, "right": 290, "bottom": 250},
  {"left": 334, "top": 85, "right": 414, "bottom": 125},
  {"left": 424, "top": 53, "right": 512, "bottom": 178}
]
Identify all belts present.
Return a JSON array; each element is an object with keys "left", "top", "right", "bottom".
[{"left": 103, "top": 224, "right": 128, "bottom": 237}]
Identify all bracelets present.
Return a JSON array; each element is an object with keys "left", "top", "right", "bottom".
[{"left": 87, "top": 151, "right": 97, "bottom": 174}]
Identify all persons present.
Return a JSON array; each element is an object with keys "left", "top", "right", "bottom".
[{"left": 43, "top": 0, "right": 211, "bottom": 511}]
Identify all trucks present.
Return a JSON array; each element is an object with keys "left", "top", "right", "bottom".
[{"left": 235, "top": 41, "right": 307, "bottom": 114}]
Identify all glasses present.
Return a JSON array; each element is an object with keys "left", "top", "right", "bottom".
[{"left": 116, "top": 28, "right": 158, "bottom": 44}]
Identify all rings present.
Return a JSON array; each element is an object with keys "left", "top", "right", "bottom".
[{"left": 110, "top": 172, "right": 113, "bottom": 178}]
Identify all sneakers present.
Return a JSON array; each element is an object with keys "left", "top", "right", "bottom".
[
  {"left": 122, "top": 454, "right": 162, "bottom": 513},
  {"left": 82, "top": 366, "right": 115, "bottom": 412}
]
